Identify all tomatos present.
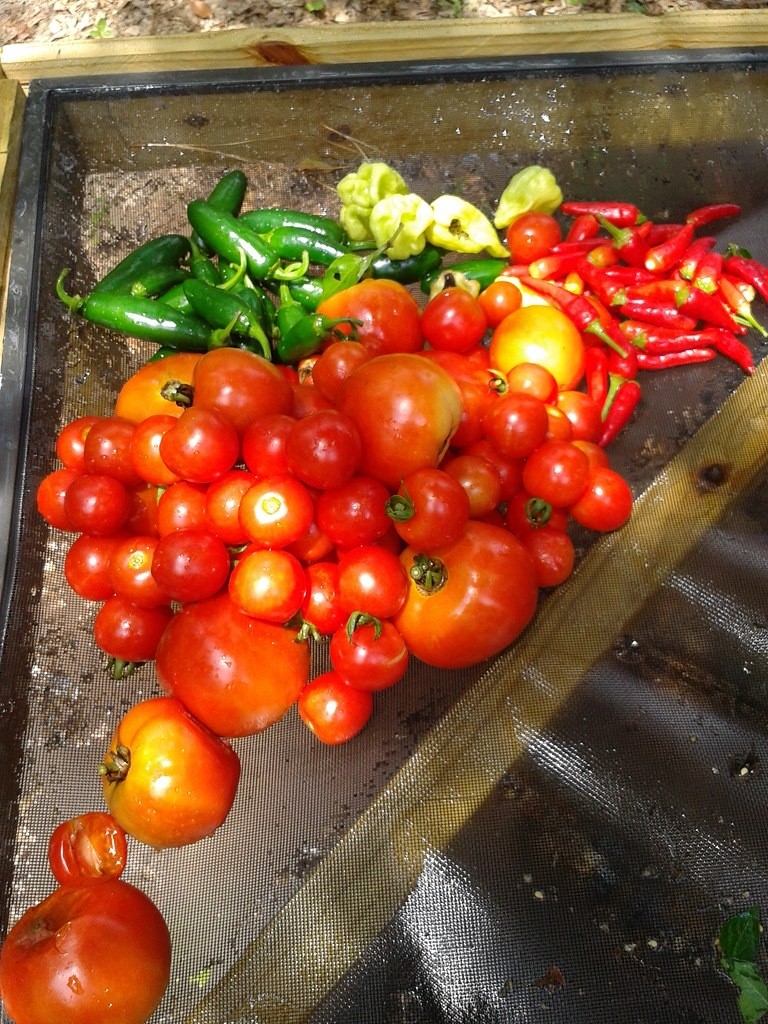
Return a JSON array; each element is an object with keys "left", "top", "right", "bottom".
[{"left": 0, "top": 282, "right": 634, "bottom": 1024}]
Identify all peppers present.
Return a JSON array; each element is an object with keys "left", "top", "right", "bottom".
[{"left": 58, "top": 162, "right": 768, "bottom": 450}]
